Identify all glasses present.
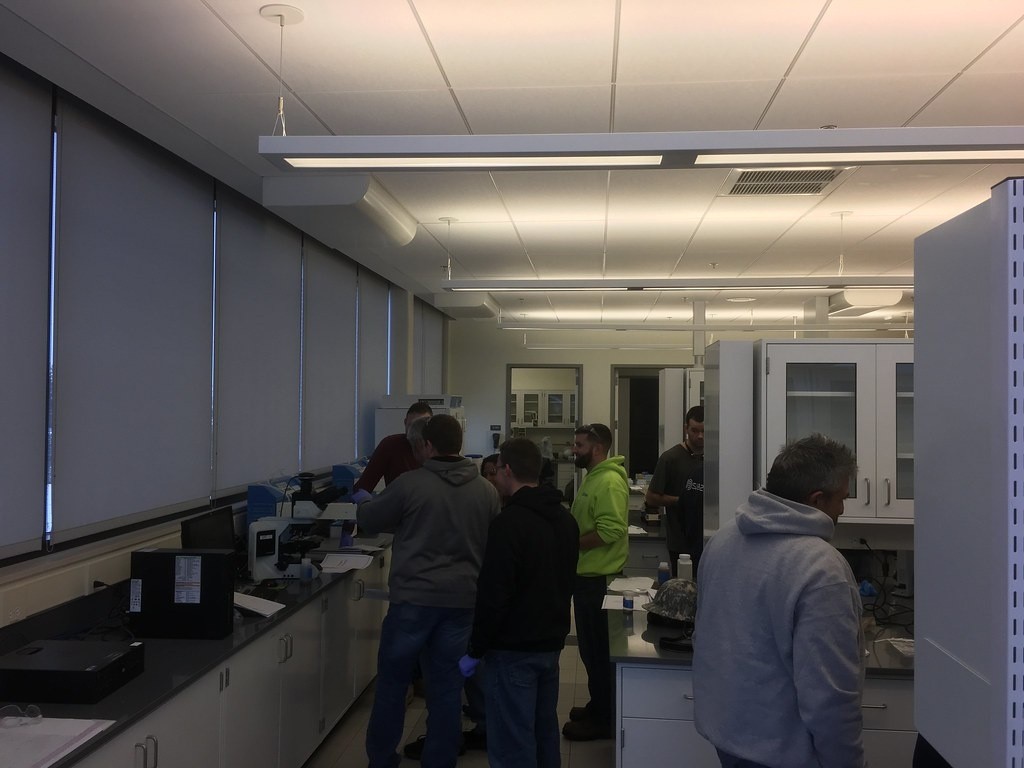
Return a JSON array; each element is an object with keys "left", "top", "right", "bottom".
[
  {"left": 491, "top": 464, "right": 513, "bottom": 474},
  {"left": 0, "top": 705, "right": 42, "bottom": 729},
  {"left": 584, "top": 424, "right": 601, "bottom": 440}
]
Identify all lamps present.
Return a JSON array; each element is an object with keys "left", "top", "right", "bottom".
[
  {"left": 253, "top": 5, "right": 1024, "bottom": 174},
  {"left": 521, "top": 332, "right": 692, "bottom": 352},
  {"left": 440, "top": 216, "right": 914, "bottom": 290},
  {"left": 494, "top": 306, "right": 916, "bottom": 331}
]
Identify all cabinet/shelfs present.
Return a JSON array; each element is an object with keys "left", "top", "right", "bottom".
[
  {"left": 615, "top": 662, "right": 721, "bottom": 768},
  {"left": 621, "top": 541, "right": 672, "bottom": 577},
  {"left": 71, "top": 545, "right": 392, "bottom": 768},
  {"left": 511, "top": 389, "right": 578, "bottom": 429},
  {"left": 684, "top": 368, "right": 705, "bottom": 410},
  {"left": 858, "top": 675, "right": 918, "bottom": 768},
  {"left": 754, "top": 337, "right": 915, "bottom": 525}
]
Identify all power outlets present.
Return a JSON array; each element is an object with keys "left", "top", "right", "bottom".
[{"left": 849, "top": 534, "right": 873, "bottom": 549}]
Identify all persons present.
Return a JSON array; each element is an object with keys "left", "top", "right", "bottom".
[
  {"left": 647, "top": 406, "right": 704, "bottom": 578},
  {"left": 691, "top": 435, "right": 867, "bottom": 768},
  {"left": 562, "top": 424, "right": 629, "bottom": 741},
  {"left": 353, "top": 403, "right": 433, "bottom": 496},
  {"left": 352, "top": 413, "right": 499, "bottom": 768},
  {"left": 405, "top": 438, "right": 579, "bottom": 768},
  {"left": 406, "top": 416, "right": 430, "bottom": 466}
]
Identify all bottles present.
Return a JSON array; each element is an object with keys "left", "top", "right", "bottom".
[
  {"left": 677, "top": 554, "right": 692, "bottom": 580},
  {"left": 622, "top": 591, "right": 633, "bottom": 614},
  {"left": 301, "top": 558, "right": 313, "bottom": 586},
  {"left": 658, "top": 561, "right": 670, "bottom": 587}
]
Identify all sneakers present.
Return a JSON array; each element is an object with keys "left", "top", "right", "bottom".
[
  {"left": 562, "top": 717, "right": 612, "bottom": 741},
  {"left": 569, "top": 706, "right": 588, "bottom": 720},
  {"left": 462, "top": 729, "right": 487, "bottom": 750},
  {"left": 404, "top": 735, "right": 466, "bottom": 759}
]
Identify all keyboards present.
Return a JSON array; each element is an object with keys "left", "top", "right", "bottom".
[{"left": 248, "top": 586, "right": 275, "bottom": 600}]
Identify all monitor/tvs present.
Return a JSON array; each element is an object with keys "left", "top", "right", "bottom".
[{"left": 181, "top": 505, "right": 237, "bottom": 560}]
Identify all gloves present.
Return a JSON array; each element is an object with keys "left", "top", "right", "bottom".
[
  {"left": 352, "top": 490, "right": 372, "bottom": 503},
  {"left": 459, "top": 654, "right": 480, "bottom": 677},
  {"left": 341, "top": 534, "right": 353, "bottom": 547}
]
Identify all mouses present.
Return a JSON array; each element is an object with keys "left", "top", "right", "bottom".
[{"left": 261, "top": 580, "right": 276, "bottom": 588}]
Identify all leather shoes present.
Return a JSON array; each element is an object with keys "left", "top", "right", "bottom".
[{"left": 660, "top": 631, "right": 694, "bottom": 653}]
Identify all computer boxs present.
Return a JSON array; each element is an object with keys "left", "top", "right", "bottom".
[
  {"left": 0, "top": 639, "right": 146, "bottom": 705},
  {"left": 131, "top": 547, "right": 235, "bottom": 640}
]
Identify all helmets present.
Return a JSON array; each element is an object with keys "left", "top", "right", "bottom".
[{"left": 642, "top": 579, "right": 698, "bottom": 624}]
